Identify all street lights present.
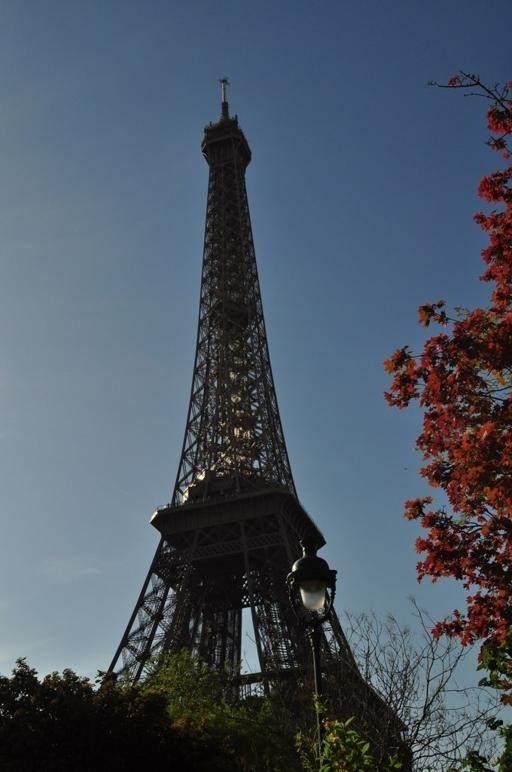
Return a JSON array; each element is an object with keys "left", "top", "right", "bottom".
[{"left": 284, "top": 537, "right": 338, "bottom": 766}]
